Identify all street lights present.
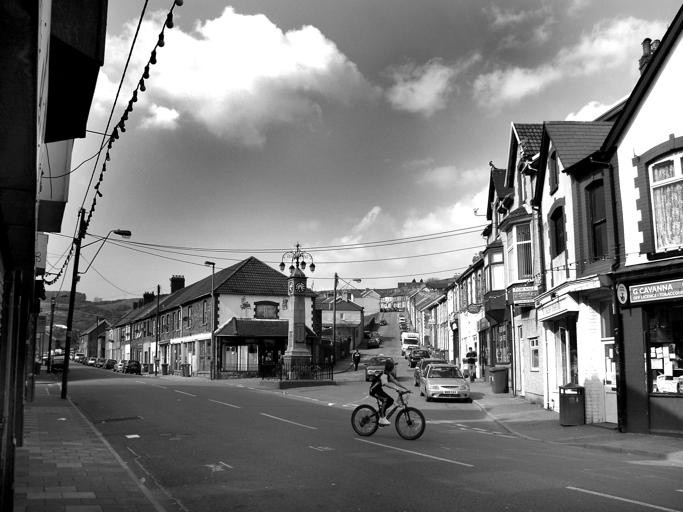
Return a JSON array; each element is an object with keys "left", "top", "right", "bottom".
[
  {"left": 203, "top": 260, "right": 216, "bottom": 377},
  {"left": 333, "top": 272, "right": 363, "bottom": 364},
  {"left": 46, "top": 293, "right": 69, "bottom": 372},
  {"left": 59, "top": 208, "right": 132, "bottom": 399}
]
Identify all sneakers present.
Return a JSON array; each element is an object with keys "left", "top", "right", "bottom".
[{"left": 379, "top": 417, "right": 391, "bottom": 425}]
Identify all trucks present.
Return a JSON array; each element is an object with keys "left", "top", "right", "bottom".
[{"left": 399, "top": 332, "right": 420, "bottom": 356}]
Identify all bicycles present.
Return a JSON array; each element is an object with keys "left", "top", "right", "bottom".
[{"left": 350, "top": 391, "right": 425, "bottom": 440}]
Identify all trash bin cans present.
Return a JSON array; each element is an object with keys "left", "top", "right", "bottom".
[
  {"left": 162, "top": 364, "right": 169, "bottom": 375},
  {"left": 559, "top": 383, "right": 586, "bottom": 426},
  {"left": 181, "top": 364, "right": 192, "bottom": 377},
  {"left": 489, "top": 367, "right": 508, "bottom": 393},
  {"left": 143, "top": 364, "right": 155, "bottom": 374}
]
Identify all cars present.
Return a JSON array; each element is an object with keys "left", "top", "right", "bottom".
[
  {"left": 42, "top": 347, "right": 141, "bottom": 375},
  {"left": 364, "top": 354, "right": 398, "bottom": 382},
  {"left": 404, "top": 346, "right": 429, "bottom": 368},
  {"left": 398, "top": 316, "right": 410, "bottom": 341},
  {"left": 418, "top": 363, "right": 470, "bottom": 402},
  {"left": 367, "top": 335, "right": 380, "bottom": 349},
  {"left": 413, "top": 358, "right": 448, "bottom": 386}
]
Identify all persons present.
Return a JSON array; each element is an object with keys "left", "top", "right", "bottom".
[
  {"left": 278, "top": 350, "right": 282, "bottom": 363},
  {"left": 369, "top": 358, "right": 409, "bottom": 429},
  {"left": 465, "top": 346, "right": 477, "bottom": 382},
  {"left": 351, "top": 348, "right": 361, "bottom": 371}
]
